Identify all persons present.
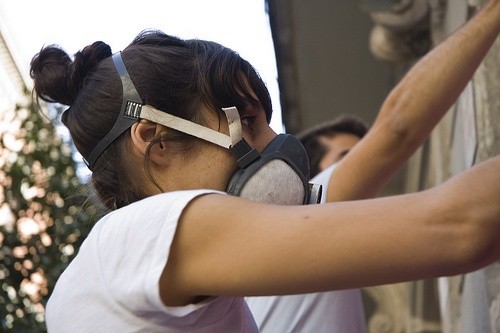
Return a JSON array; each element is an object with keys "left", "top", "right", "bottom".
[
  {"left": 30, "top": 0, "right": 500, "bottom": 332},
  {"left": 244, "top": 2, "right": 498, "bottom": 331}
]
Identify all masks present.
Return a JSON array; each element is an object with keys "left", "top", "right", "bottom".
[{"left": 226, "top": 134, "right": 322, "bottom": 205}]
